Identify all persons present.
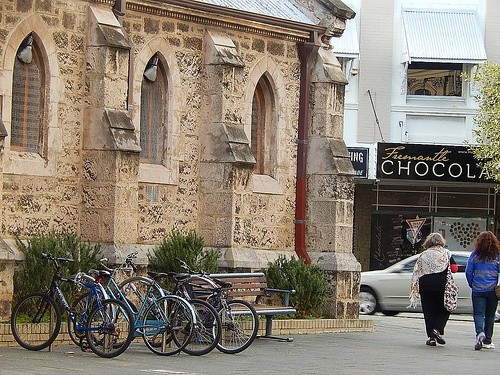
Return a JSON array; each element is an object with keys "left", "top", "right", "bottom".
[
  {"left": 411, "top": 233, "right": 458, "bottom": 346},
  {"left": 465, "top": 231, "right": 500, "bottom": 350}
]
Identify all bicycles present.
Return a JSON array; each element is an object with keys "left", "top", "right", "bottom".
[
  {"left": 67, "top": 251, "right": 260, "bottom": 357},
  {"left": 11, "top": 253, "right": 135, "bottom": 359}
]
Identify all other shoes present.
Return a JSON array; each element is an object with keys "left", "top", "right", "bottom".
[
  {"left": 482, "top": 343, "right": 495, "bottom": 349},
  {"left": 474, "top": 332, "right": 486, "bottom": 350},
  {"left": 426, "top": 338, "right": 436, "bottom": 346},
  {"left": 431, "top": 329, "right": 445, "bottom": 344}
]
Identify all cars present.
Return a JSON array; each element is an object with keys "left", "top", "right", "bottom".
[{"left": 357, "top": 249, "right": 500, "bottom": 320}]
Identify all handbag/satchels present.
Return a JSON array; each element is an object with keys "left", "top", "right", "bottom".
[
  {"left": 495, "top": 285, "right": 500, "bottom": 300},
  {"left": 444, "top": 268, "right": 459, "bottom": 313}
]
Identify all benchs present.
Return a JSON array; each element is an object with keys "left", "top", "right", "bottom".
[{"left": 186, "top": 271, "right": 296, "bottom": 345}]
